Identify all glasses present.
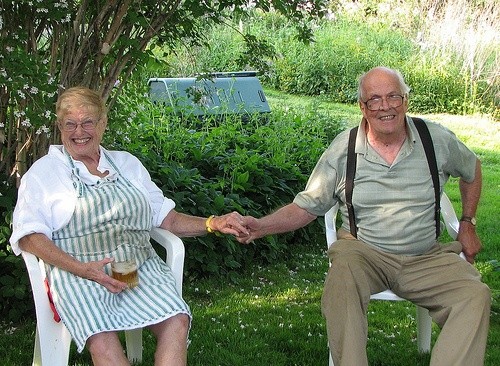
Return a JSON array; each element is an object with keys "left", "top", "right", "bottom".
[
  {"left": 57, "top": 118, "right": 101, "bottom": 132},
  {"left": 360, "top": 93, "right": 408, "bottom": 111}
]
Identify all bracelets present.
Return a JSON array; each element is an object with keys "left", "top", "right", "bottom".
[{"left": 205, "top": 213, "right": 218, "bottom": 233}]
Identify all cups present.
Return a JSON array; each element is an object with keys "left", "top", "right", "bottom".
[{"left": 111, "top": 251, "right": 139, "bottom": 287}]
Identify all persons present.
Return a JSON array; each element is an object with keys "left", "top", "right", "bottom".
[
  {"left": 10, "top": 86, "right": 250, "bottom": 366},
  {"left": 230, "top": 66, "right": 492, "bottom": 366}
]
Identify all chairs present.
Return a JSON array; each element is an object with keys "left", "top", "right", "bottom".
[
  {"left": 324, "top": 192, "right": 466, "bottom": 366},
  {"left": 21, "top": 227, "right": 184, "bottom": 366}
]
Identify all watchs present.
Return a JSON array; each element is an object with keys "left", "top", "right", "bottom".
[{"left": 461, "top": 216, "right": 477, "bottom": 226}]
